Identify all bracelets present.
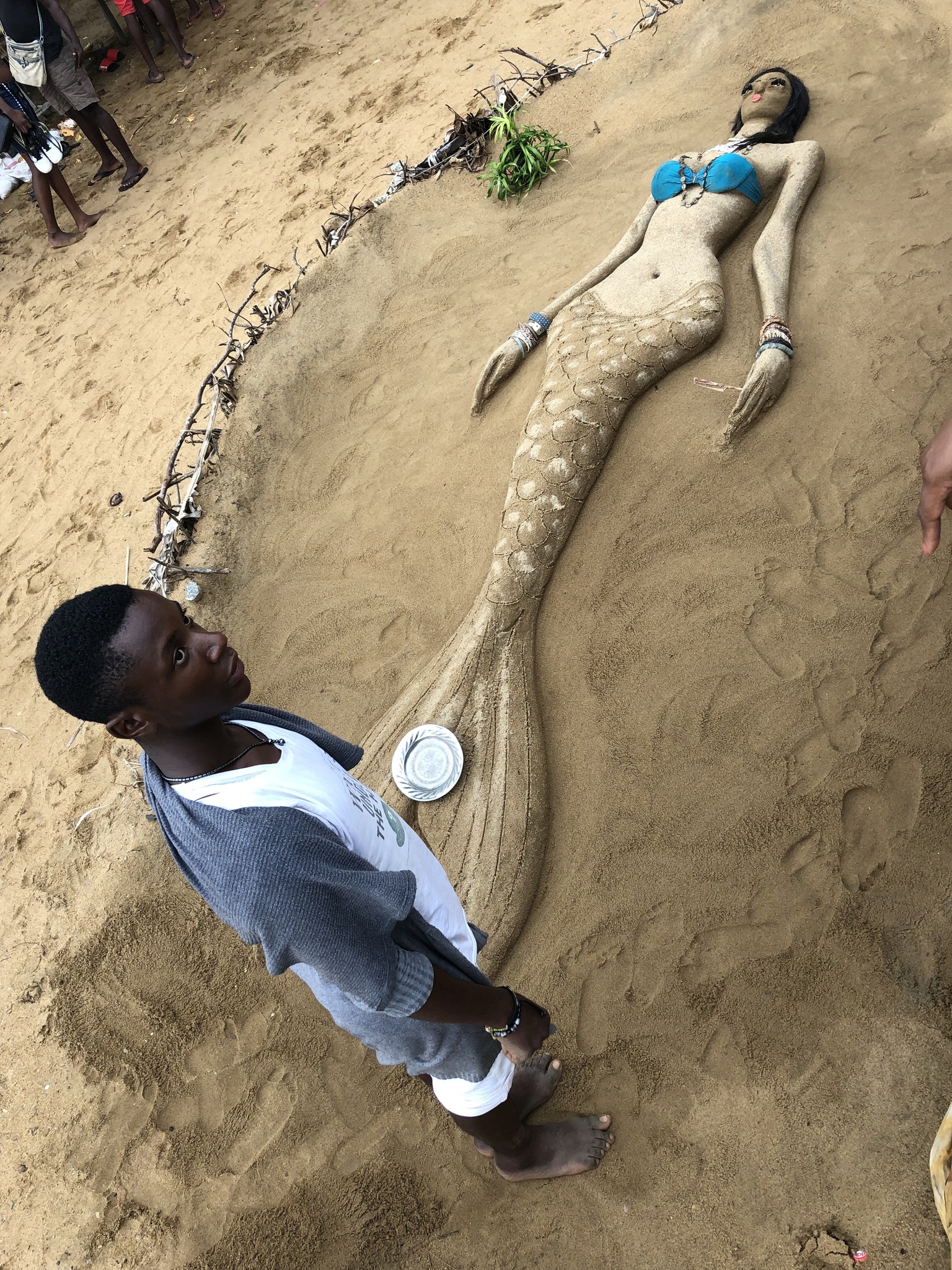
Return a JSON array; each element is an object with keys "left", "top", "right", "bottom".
[
  {"left": 754, "top": 314, "right": 795, "bottom": 359},
  {"left": 508, "top": 311, "right": 552, "bottom": 359},
  {"left": 485, "top": 985, "right": 522, "bottom": 1040}
]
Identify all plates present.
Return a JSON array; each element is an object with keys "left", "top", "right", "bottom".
[{"left": 393, "top": 725, "right": 463, "bottom": 801}]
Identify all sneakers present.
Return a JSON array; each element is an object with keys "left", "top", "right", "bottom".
[{"left": 19, "top": 122, "right": 63, "bottom": 174}]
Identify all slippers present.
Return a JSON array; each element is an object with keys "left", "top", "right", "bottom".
[
  {"left": 185, "top": 9, "right": 201, "bottom": 29},
  {"left": 211, "top": 4, "right": 225, "bottom": 20},
  {"left": 59, "top": 138, "right": 80, "bottom": 159},
  {"left": 88, "top": 163, "right": 123, "bottom": 186},
  {"left": 93, "top": 47, "right": 126, "bottom": 73},
  {"left": 145, "top": 69, "right": 165, "bottom": 83},
  {"left": 184, "top": 53, "right": 194, "bottom": 69},
  {"left": 118, "top": 166, "right": 148, "bottom": 192}
]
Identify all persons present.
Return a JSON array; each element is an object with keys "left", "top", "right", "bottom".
[
  {"left": 36, "top": 585, "right": 620, "bottom": 1181},
  {"left": 916, "top": 413, "right": 952, "bottom": 557},
  {"left": 113, "top": 0, "right": 194, "bottom": 84},
  {"left": 0, "top": 0, "right": 149, "bottom": 192},
  {"left": 470, "top": 66, "right": 825, "bottom": 438},
  {"left": 185, "top": 0, "right": 225, "bottom": 29},
  {"left": 0, "top": 57, "right": 107, "bottom": 248}
]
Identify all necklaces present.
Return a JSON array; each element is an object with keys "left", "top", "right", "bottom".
[
  {"left": 678, "top": 142, "right": 757, "bottom": 209},
  {"left": 157, "top": 720, "right": 288, "bottom": 782}
]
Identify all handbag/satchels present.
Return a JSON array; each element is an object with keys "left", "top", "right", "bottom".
[
  {"left": 0, "top": 152, "right": 34, "bottom": 199},
  {"left": 0, "top": 35, "right": 49, "bottom": 86}
]
